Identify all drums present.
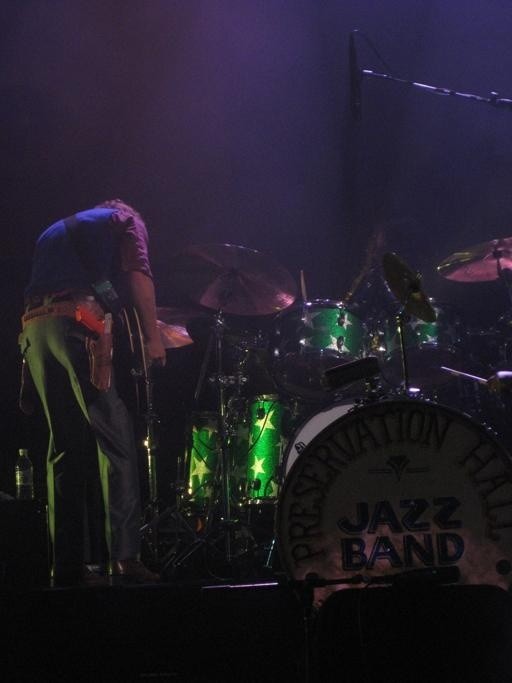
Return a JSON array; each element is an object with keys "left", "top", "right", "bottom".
[
  {"left": 271, "top": 297, "right": 370, "bottom": 402},
  {"left": 366, "top": 314, "right": 448, "bottom": 386},
  {"left": 274, "top": 403, "right": 512, "bottom": 635},
  {"left": 177, "top": 393, "right": 280, "bottom": 522}
]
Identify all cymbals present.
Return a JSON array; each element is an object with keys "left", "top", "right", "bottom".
[
  {"left": 164, "top": 245, "right": 298, "bottom": 322},
  {"left": 438, "top": 238, "right": 512, "bottom": 284},
  {"left": 382, "top": 254, "right": 436, "bottom": 323},
  {"left": 157, "top": 307, "right": 214, "bottom": 349}
]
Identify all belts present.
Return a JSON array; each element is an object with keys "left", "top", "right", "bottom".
[{"left": 21, "top": 302, "right": 76, "bottom": 328}]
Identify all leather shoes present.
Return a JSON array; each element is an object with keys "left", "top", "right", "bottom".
[{"left": 72, "top": 560, "right": 161, "bottom": 587}]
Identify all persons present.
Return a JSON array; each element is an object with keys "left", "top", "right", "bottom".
[
  {"left": 432, "top": 371, "right": 473, "bottom": 414},
  {"left": 487, "top": 370, "right": 512, "bottom": 394},
  {"left": 18, "top": 198, "right": 167, "bottom": 586}
]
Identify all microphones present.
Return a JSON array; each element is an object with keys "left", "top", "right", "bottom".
[{"left": 439, "top": 366, "right": 487, "bottom": 384}]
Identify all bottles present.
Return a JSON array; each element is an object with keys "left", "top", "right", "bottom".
[{"left": 15, "top": 447, "right": 34, "bottom": 499}]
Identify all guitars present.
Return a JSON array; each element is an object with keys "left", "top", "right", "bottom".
[{"left": 121, "top": 305, "right": 149, "bottom": 415}]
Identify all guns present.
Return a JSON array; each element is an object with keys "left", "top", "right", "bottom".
[{"left": 73, "top": 306, "right": 111, "bottom": 335}]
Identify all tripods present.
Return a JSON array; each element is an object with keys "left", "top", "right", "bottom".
[{"left": 140, "top": 310, "right": 275, "bottom": 573}]
